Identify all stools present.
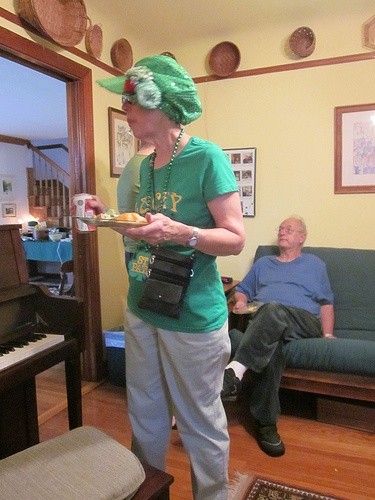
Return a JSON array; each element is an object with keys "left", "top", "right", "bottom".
[{"left": 0, "top": 424, "right": 173, "bottom": 500}]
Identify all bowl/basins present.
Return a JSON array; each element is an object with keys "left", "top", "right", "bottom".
[{"left": 48, "top": 232, "right": 63, "bottom": 241}]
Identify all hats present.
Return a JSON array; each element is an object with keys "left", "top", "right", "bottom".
[{"left": 96, "top": 55, "right": 203, "bottom": 126}]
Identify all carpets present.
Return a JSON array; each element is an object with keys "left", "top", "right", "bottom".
[{"left": 226, "top": 471, "right": 345, "bottom": 500}]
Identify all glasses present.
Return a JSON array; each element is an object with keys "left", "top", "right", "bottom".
[
  {"left": 275, "top": 227, "right": 301, "bottom": 234},
  {"left": 120, "top": 91, "right": 139, "bottom": 106}
]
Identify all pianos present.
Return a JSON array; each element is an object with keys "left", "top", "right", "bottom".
[{"left": 0, "top": 224, "right": 88, "bottom": 460}]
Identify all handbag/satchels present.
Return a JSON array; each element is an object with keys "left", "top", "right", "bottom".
[{"left": 139, "top": 249, "right": 197, "bottom": 318}]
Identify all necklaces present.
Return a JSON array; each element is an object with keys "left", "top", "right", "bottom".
[{"left": 147, "top": 129, "right": 184, "bottom": 215}]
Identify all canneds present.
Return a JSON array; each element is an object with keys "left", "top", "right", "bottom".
[{"left": 73, "top": 193, "right": 96, "bottom": 233}]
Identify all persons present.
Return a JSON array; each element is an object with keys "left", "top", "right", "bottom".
[
  {"left": 220, "top": 212, "right": 337, "bottom": 457},
  {"left": 116, "top": 140, "right": 155, "bottom": 271},
  {"left": 87, "top": 56, "right": 246, "bottom": 500}
]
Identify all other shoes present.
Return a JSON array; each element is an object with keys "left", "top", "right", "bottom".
[
  {"left": 220, "top": 369, "right": 241, "bottom": 401},
  {"left": 257, "top": 424, "right": 285, "bottom": 457}
]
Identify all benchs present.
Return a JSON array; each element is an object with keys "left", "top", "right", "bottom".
[{"left": 251, "top": 246, "right": 375, "bottom": 403}]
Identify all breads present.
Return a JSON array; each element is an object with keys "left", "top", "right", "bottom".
[{"left": 113, "top": 212, "right": 147, "bottom": 223}]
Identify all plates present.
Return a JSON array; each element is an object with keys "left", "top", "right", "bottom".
[
  {"left": 75, "top": 217, "right": 149, "bottom": 227},
  {"left": 232, "top": 302, "right": 265, "bottom": 314}
]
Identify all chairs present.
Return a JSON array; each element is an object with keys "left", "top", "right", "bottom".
[{"left": 58, "top": 260, "right": 75, "bottom": 295}]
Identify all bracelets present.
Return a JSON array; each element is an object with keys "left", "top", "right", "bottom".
[{"left": 323, "top": 333, "right": 334, "bottom": 338}]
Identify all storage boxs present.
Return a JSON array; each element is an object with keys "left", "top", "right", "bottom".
[{"left": 103, "top": 324, "right": 127, "bottom": 388}]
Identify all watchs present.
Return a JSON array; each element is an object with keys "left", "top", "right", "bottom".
[{"left": 184, "top": 227, "right": 198, "bottom": 248}]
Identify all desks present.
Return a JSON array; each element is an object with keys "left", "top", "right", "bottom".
[{"left": 22, "top": 238, "right": 73, "bottom": 286}]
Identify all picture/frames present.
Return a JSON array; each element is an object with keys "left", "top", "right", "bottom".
[
  {"left": 221, "top": 147, "right": 256, "bottom": 218},
  {"left": 0, "top": 174, "right": 17, "bottom": 202},
  {"left": 1, "top": 203, "right": 17, "bottom": 217},
  {"left": 333, "top": 103, "right": 375, "bottom": 195},
  {"left": 108, "top": 107, "right": 142, "bottom": 178}
]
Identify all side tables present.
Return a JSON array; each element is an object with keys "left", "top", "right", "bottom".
[{"left": 222, "top": 280, "right": 241, "bottom": 314}]
